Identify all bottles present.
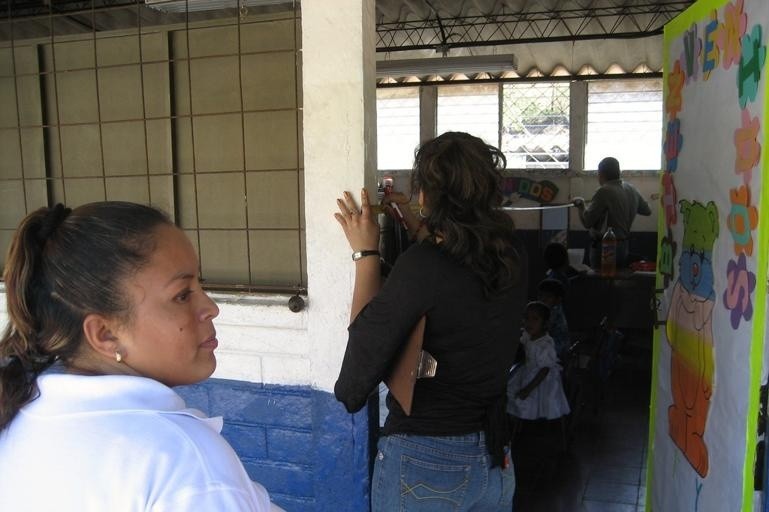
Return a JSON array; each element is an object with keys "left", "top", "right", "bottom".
[{"left": 602, "top": 225, "right": 618, "bottom": 275}]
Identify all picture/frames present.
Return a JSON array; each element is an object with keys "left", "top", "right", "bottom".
[{"left": 540, "top": 203, "right": 569, "bottom": 249}]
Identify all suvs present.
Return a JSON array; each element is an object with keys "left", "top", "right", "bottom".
[{"left": 500, "top": 114, "right": 570, "bottom": 162}]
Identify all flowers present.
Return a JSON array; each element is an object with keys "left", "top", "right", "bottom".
[{"left": 659, "top": 0, "right": 766, "bottom": 329}]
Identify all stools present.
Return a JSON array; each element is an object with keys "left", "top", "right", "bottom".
[{"left": 583, "top": 317, "right": 624, "bottom": 416}]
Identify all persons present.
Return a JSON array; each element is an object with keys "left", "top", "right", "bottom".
[
  {"left": 508, "top": 300, "right": 572, "bottom": 433},
  {"left": 332, "top": 131, "right": 532, "bottom": 512},
  {"left": 534, "top": 280, "right": 572, "bottom": 371},
  {"left": 571, "top": 156, "right": 654, "bottom": 272},
  {"left": 0, "top": 201, "right": 291, "bottom": 512},
  {"left": 540, "top": 239, "right": 579, "bottom": 287}
]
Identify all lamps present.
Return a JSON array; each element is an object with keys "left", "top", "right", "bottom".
[{"left": 374, "top": 44, "right": 517, "bottom": 78}]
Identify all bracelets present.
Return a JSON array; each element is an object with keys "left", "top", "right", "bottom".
[
  {"left": 351, "top": 248, "right": 382, "bottom": 261},
  {"left": 407, "top": 219, "right": 426, "bottom": 243}
]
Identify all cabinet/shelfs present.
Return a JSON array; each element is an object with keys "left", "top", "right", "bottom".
[{"left": 587, "top": 271, "right": 656, "bottom": 329}]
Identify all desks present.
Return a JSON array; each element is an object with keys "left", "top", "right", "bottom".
[{"left": 492, "top": 203, "right": 591, "bottom": 231}]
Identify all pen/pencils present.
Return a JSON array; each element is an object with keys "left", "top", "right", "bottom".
[{"left": 383, "top": 188, "right": 408, "bottom": 231}]
